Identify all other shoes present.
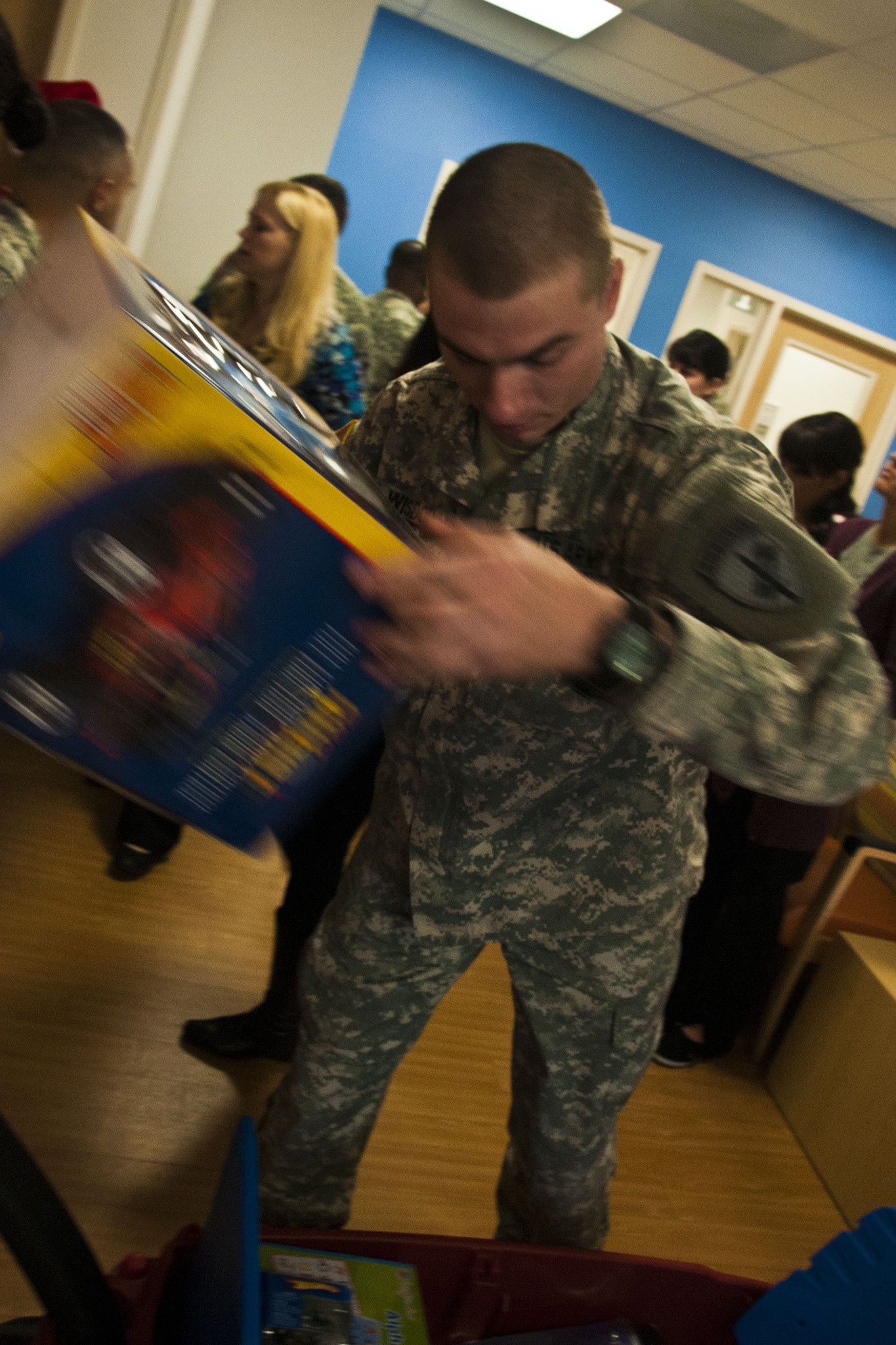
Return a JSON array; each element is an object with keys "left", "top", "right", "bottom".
[
  {"left": 183, "top": 1000, "right": 298, "bottom": 1063},
  {"left": 649, "top": 1025, "right": 713, "bottom": 1067},
  {"left": 109, "top": 843, "right": 158, "bottom": 881}
]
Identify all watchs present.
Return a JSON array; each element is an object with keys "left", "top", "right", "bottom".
[{"left": 562, "top": 588, "right": 668, "bottom": 702}]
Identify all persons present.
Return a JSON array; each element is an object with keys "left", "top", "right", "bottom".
[
  {"left": 247, "top": 144, "right": 891, "bottom": 1252},
  {"left": 667, "top": 330, "right": 731, "bottom": 403},
  {"left": 652, "top": 413, "right": 896, "bottom": 1070},
  {"left": 45, "top": 98, "right": 134, "bottom": 229},
  {"left": 88, "top": 176, "right": 444, "bottom": 1059}
]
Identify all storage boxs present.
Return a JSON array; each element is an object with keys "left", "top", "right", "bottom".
[{"left": 1, "top": 203, "right": 438, "bottom": 860}]
